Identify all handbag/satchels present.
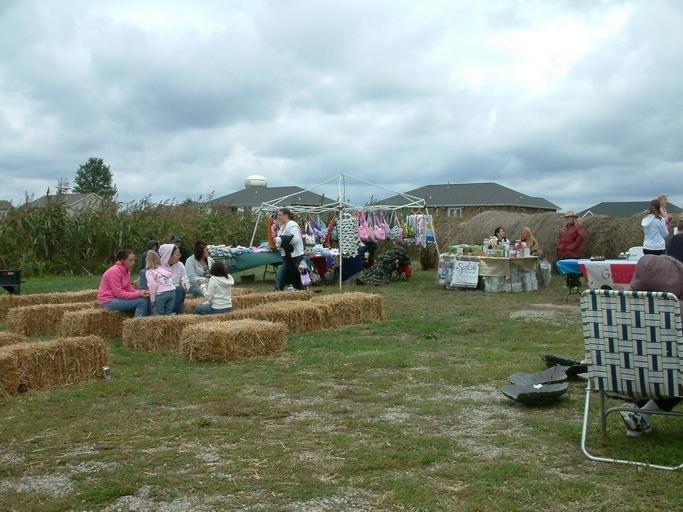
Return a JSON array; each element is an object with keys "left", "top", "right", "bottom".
[
  {"left": 301, "top": 214, "right": 338, "bottom": 246},
  {"left": 356, "top": 210, "right": 417, "bottom": 243}
]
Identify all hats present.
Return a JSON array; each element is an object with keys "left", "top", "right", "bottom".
[
  {"left": 560, "top": 211, "right": 579, "bottom": 219},
  {"left": 171, "top": 234, "right": 183, "bottom": 242}
]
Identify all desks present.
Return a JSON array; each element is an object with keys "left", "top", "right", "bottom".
[
  {"left": 202, "top": 241, "right": 378, "bottom": 286},
  {"left": 436, "top": 252, "right": 538, "bottom": 295},
  {"left": 554, "top": 258, "right": 639, "bottom": 304}
]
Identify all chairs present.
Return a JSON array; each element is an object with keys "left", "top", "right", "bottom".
[
  {"left": 575, "top": 288, "right": 683, "bottom": 473},
  {"left": 385, "top": 261, "right": 409, "bottom": 283}
]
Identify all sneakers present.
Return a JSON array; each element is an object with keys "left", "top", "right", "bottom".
[
  {"left": 626, "top": 424, "right": 653, "bottom": 437},
  {"left": 620, "top": 404, "right": 647, "bottom": 432}
]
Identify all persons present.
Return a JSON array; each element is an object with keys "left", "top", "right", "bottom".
[
  {"left": 96, "top": 234, "right": 234, "bottom": 318},
  {"left": 667, "top": 216, "right": 683, "bottom": 262},
  {"left": 641, "top": 199, "right": 669, "bottom": 255},
  {"left": 619, "top": 254, "right": 683, "bottom": 437},
  {"left": 488, "top": 227, "right": 509, "bottom": 249},
  {"left": 520, "top": 226, "right": 539, "bottom": 256},
  {"left": 657, "top": 194, "right": 668, "bottom": 225},
  {"left": 557, "top": 211, "right": 586, "bottom": 290},
  {"left": 272, "top": 208, "right": 305, "bottom": 291}
]
